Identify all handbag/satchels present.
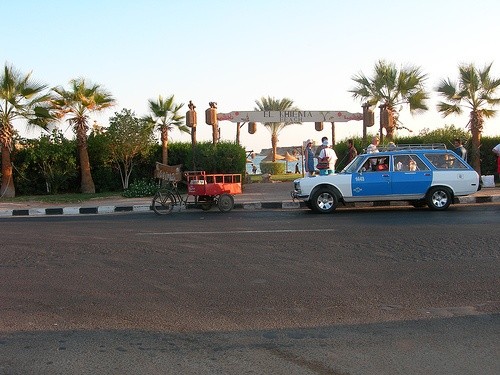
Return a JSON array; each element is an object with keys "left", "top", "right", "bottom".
[
  {"left": 305, "top": 169, "right": 311, "bottom": 177},
  {"left": 316, "top": 148, "right": 330, "bottom": 169}
]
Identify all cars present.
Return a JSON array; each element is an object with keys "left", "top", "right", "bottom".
[{"left": 289, "top": 142, "right": 480, "bottom": 214}]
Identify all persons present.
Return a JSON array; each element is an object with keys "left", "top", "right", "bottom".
[
  {"left": 345, "top": 139, "right": 357, "bottom": 166},
  {"left": 315, "top": 137, "right": 328, "bottom": 160},
  {"left": 251, "top": 164, "right": 257, "bottom": 174},
  {"left": 318, "top": 140, "right": 338, "bottom": 176},
  {"left": 492, "top": 143, "right": 500, "bottom": 178},
  {"left": 362, "top": 137, "right": 467, "bottom": 172},
  {"left": 295, "top": 163, "right": 300, "bottom": 174},
  {"left": 304, "top": 141, "right": 314, "bottom": 175}
]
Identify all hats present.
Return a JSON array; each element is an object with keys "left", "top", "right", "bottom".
[
  {"left": 323, "top": 140, "right": 330, "bottom": 146},
  {"left": 306, "top": 139, "right": 312, "bottom": 146},
  {"left": 374, "top": 137, "right": 379, "bottom": 141}
]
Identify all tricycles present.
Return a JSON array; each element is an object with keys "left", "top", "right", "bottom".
[{"left": 152, "top": 161, "right": 243, "bottom": 215}]
